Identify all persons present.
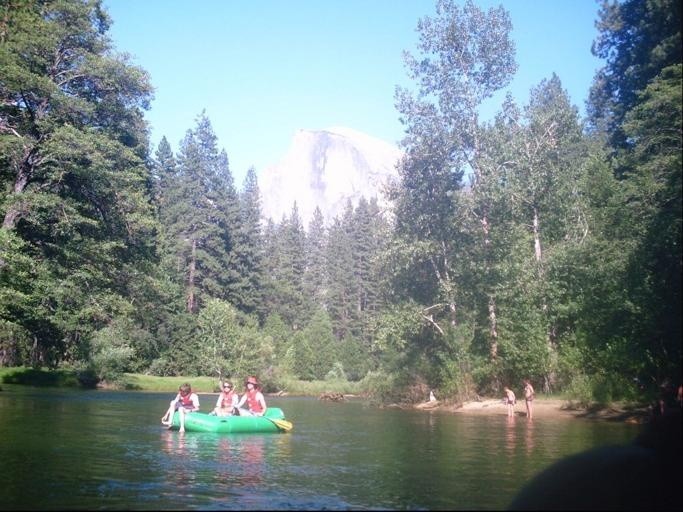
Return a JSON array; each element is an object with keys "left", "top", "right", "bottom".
[
  {"left": 162, "top": 383, "right": 202, "bottom": 434},
  {"left": 503, "top": 386, "right": 516, "bottom": 418},
  {"left": 523, "top": 380, "right": 535, "bottom": 419},
  {"left": 233, "top": 376, "right": 266, "bottom": 417},
  {"left": 208, "top": 381, "right": 239, "bottom": 416}
]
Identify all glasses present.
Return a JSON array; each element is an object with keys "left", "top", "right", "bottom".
[{"left": 223, "top": 386, "right": 230, "bottom": 388}]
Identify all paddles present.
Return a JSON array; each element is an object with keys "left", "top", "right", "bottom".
[{"left": 250, "top": 411, "right": 292, "bottom": 431}]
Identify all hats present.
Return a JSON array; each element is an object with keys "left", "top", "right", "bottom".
[{"left": 247, "top": 376, "right": 258, "bottom": 384}]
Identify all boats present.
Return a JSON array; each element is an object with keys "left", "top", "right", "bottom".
[{"left": 167, "top": 407, "right": 285, "bottom": 433}]
